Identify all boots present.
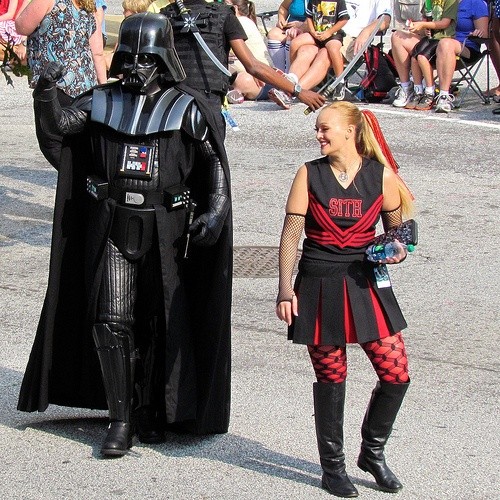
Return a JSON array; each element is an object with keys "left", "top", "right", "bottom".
[
  {"left": 133, "top": 333, "right": 168, "bottom": 442},
  {"left": 358, "top": 376, "right": 410, "bottom": 492},
  {"left": 91, "top": 323, "right": 134, "bottom": 455},
  {"left": 312, "top": 380, "right": 359, "bottom": 498}
]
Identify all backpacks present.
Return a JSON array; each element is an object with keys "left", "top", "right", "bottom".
[{"left": 353, "top": 42, "right": 398, "bottom": 104}]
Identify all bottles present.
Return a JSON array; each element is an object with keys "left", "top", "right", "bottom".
[
  {"left": 366, "top": 242, "right": 415, "bottom": 262},
  {"left": 425, "top": 10, "right": 434, "bottom": 38}
]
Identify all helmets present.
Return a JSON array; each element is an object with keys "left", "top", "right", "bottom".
[{"left": 108, "top": 11, "right": 187, "bottom": 83}]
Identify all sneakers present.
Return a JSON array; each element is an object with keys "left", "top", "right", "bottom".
[
  {"left": 416, "top": 91, "right": 437, "bottom": 110},
  {"left": 392, "top": 82, "right": 414, "bottom": 107},
  {"left": 332, "top": 81, "right": 346, "bottom": 100},
  {"left": 267, "top": 88, "right": 292, "bottom": 109},
  {"left": 434, "top": 93, "right": 456, "bottom": 113},
  {"left": 405, "top": 86, "right": 423, "bottom": 108}
]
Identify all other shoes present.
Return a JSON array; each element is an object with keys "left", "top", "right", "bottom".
[
  {"left": 492, "top": 108, "right": 500, "bottom": 115},
  {"left": 493, "top": 95, "right": 500, "bottom": 103},
  {"left": 483, "top": 87, "right": 500, "bottom": 97},
  {"left": 226, "top": 89, "right": 244, "bottom": 104}
]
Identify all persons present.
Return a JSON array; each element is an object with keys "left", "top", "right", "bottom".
[
  {"left": 15, "top": 0, "right": 500, "bottom": 115},
  {"left": 274, "top": 102, "right": 414, "bottom": 498},
  {"left": 159, "top": 0, "right": 326, "bottom": 143},
  {"left": 14, "top": 12, "right": 235, "bottom": 458}
]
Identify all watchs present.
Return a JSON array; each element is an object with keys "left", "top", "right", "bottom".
[{"left": 291, "top": 84, "right": 302, "bottom": 97}]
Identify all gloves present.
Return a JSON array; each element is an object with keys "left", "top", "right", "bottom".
[
  {"left": 32, "top": 62, "right": 67, "bottom": 102},
  {"left": 189, "top": 195, "right": 231, "bottom": 246}
]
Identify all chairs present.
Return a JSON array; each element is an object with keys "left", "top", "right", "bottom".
[{"left": 256, "top": 0, "right": 491, "bottom": 109}]
